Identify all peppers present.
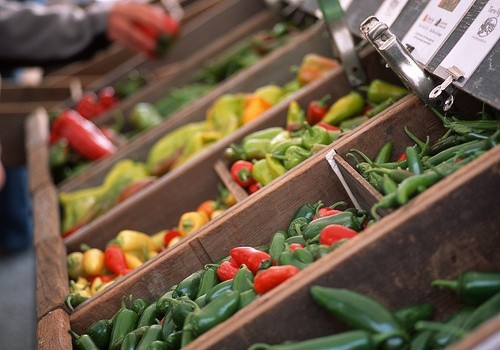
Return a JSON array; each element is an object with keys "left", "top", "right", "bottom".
[{"left": 48, "top": 2, "right": 500, "bottom": 350}]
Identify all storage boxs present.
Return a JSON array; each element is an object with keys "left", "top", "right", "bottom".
[{"left": 0, "top": 0, "right": 500, "bottom": 350}]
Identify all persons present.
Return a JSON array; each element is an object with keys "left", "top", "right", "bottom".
[{"left": 0, "top": 0, "right": 185, "bottom": 257}]
